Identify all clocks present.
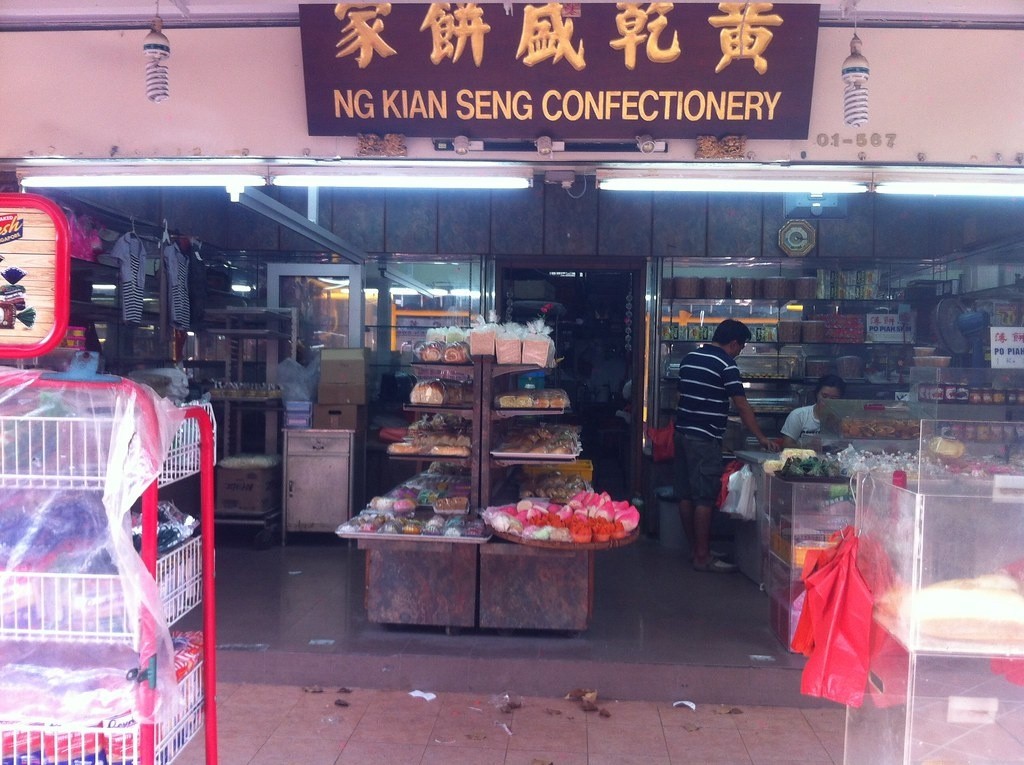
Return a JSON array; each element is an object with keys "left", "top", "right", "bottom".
[{"left": 778, "top": 219, "right": 816, "bottom": 257}]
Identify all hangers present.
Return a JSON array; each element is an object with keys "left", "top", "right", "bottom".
[
  {"left": 170, "top": 229, "right": 187, "bottom": 260},
  {"left": 127, "top": 215, "right": 139, "bottom": 239},
  {"left": 158, "top": 219, "right": 171, "bottom": 249}
]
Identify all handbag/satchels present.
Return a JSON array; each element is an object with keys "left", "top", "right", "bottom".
[
  {"left": 790, "top": 528, "right": 908, "bottom": 711},
  {"left": 714, "top": 462, "right": 758, "bottom": 521},
  {"left": 647, "top": 416, "right": 675, "bottom": 463}
]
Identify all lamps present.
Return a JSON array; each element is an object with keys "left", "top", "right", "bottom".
[
  {"left": 536, "top": 135, "right": 551, "bottom": 156},
  {"left": 15, "top": 165, "right": 534, "bottom": 190},
  {"left": 636, "top": 135, "right": 654, "bottom": 154},
  {"left": 595, "top": 163, "right": 1024, "bottom": 197},
  {"left": 452, "top": 135, "right": 468, "bottom": 155}
]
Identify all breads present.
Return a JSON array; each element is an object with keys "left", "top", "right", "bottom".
[
  {"left": 518, "top": 470, "right": 589, "bottom": 505},
  {"left": 337, "top": 459, "right": 485, "bottom": 537},
  {"left": 387, "top": 325, "right": 578, "bottom": 457}
]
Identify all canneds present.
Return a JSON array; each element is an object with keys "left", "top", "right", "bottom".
[{"left": 918, "top": 380, "right": 1024, "bottom": 443}]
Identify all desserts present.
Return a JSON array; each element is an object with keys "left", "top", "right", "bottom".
[{"left": 483, "top": 488, "right": 640, "bottom": 543}]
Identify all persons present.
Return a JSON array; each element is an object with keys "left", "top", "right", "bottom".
[
  {"left": 582, "top": 360, "right": 606, "bottom": 395},
  {"left": 779, "top": 373, "right": 847, "bottom": 448},
  {"left": 672, "top": 318, "right": 779, "bottom": 573}
]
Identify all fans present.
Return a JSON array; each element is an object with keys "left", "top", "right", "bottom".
[{"left": 932, "top": 299, "right": 991, "bottom": 391}]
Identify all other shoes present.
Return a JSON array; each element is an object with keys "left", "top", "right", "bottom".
[
  {"left": 694, "top": 556, "right": 738, "bottom": 573},
  {"left": 688, "top": 549, "right": 732, "bottom": 563}
]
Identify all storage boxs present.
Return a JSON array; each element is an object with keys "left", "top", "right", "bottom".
[
  {"left": 521, "top": 460, "right": 592, "bottom": 482},
  {"left": 0, "top": 327, "right": 97, "bottom": 373},
  {"left": 313, "top": 348, "right": 372, "bottom": 430},
  {"left": 216, "top": 455, "right": 282, "bottom": 515},
  {"left": 769, "top": 271, "right": 1023, "bottom": 765}
]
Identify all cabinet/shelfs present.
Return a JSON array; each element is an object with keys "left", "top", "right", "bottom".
[
  {"left": 651, "top": 231, "right": 1024, "bottom": 467},
  {"left": 0, "top": 364, "right": 218, "bottom": 765},
  {"left": 69, "top": 256, "right": 173, "bottom": 374},
  {"left": 356, "top": 354, "right": 594, "bottom": 636},
  {"left": 280, "top": 427, "right": 368, "bottom": 547}
]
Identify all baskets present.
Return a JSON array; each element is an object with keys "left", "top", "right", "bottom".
[
  {"left": 0, "top": 658, "right": 205, "bottom": 765},
  {"left": 0, "top": 536, "right": 216, "bottom": 647},
  {"left": 1, "top": 400, "right": 218, "bottom": 496}
]
